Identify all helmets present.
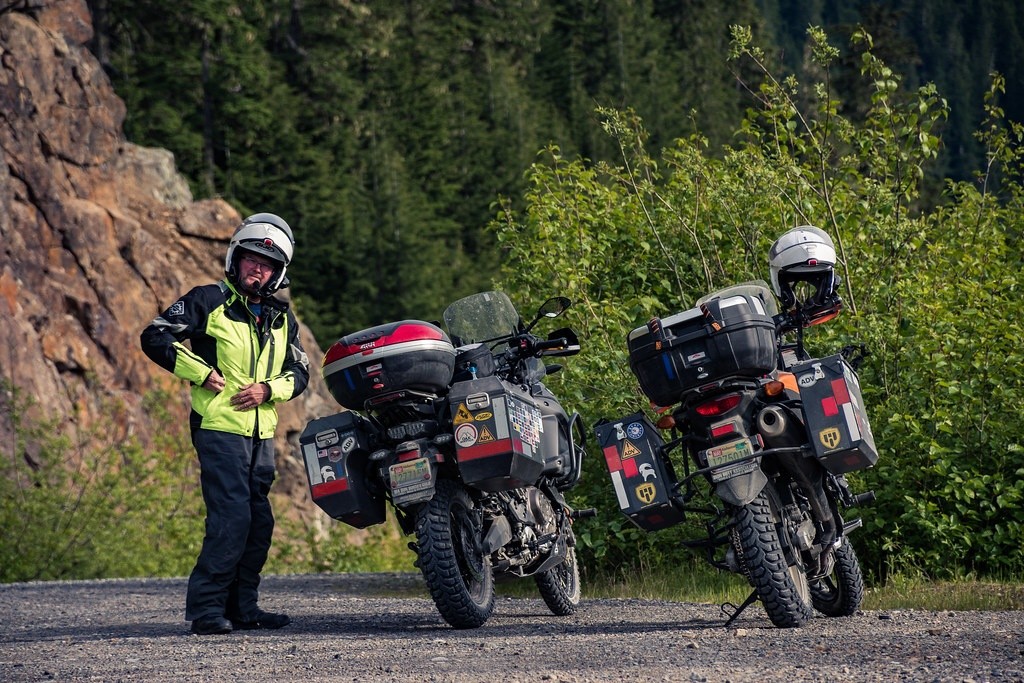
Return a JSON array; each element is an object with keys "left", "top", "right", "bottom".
[
  {"left": 225, "top": 212, "right": 295, "bottom": 296},
  {"left": 768, "top": 225, "right": 837, "bottom": 309}
]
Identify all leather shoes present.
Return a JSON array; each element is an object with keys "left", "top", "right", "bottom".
[
  {"left": 190, "top": 613, "right": 233, "bottom": 635},
  {"left": 228, "top": 603, "right": 292, "bottom": 630}
]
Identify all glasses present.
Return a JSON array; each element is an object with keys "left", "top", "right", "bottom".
[{"left": 242, "top": 255, "right": 275, "bottom": 271}]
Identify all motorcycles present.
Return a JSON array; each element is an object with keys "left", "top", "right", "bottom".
[
  {"left": 592, "top": 279, "right": 880, "bottom": 630},
  {"left": 299, "top": 290, "right": 599, "bottom": 629}
]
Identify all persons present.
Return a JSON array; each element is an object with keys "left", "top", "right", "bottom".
[{"left": 139, "top": 213, "right": 311, "bottom": 634}]
